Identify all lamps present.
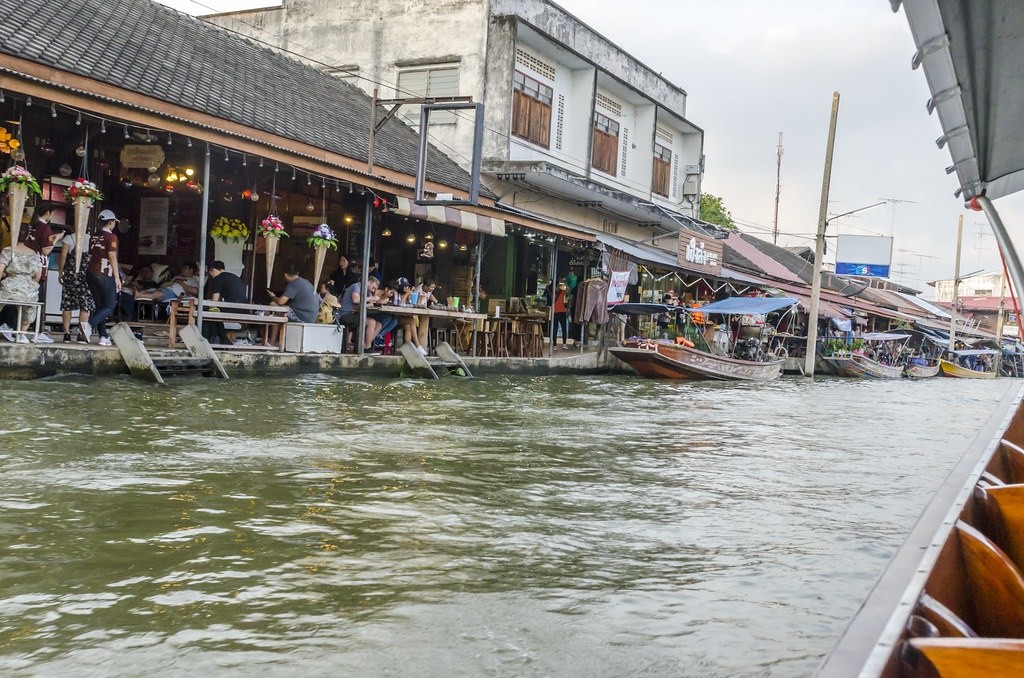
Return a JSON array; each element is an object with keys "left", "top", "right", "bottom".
[
  {"left": 166, "top": 168, "right": 187, "bottom": 181},
  {"left": 438, "top": 239, "right": 447, "bottom": 247},
  {"left": 382, "top": 213, "right": 391, "bottom": 236},
  {"left": 406, "top": 219, "right": 415, "bottom": 241},
  {"left": 459, "top": 229, "right": 468, "bottom": 251}
]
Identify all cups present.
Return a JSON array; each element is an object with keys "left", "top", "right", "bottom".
[
  {"left": 420, "top": 293, "right": 427, "bottom": 308},
  {"left": 495, "top": 305, "right": 500, "bottom": 317},
  {"left": 453, "top": 297, "right": 459, "bottom": 308},
  {"left": 447, "top": 295, "right": 453, "bottom": 309},
  {"left": 411, "top": 292, "right": 418, "bottom": 305}
]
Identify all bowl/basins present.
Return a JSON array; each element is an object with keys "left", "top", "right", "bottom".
[{"left": 373, "top": 302, "right": 381, "bottom": 307}]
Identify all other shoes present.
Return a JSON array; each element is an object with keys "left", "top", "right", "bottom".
[
  {"left": 373, "top": 334, "right": 386, "bottom": 347},
  {"left": 573, "top": 340, "right": 581, "bottom": 347},
  {"left": 553, "top": 346, "right": 559, "bottom": 350},
  {"left": 417, "top": 345, "right": 428, "bottom": 355},
  {"left": 363, "top": 347, "right": 380, "bottom": 355},
  {"left": 563, "top": 344, "right": 568, "bottom": 350},
  {"left": 154, "top": 330, "right": 170, "bottom": 337}
]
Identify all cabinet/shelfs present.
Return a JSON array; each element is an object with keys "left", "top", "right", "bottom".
[{"left": 33, "top": 176, "right": 96, "bottom": 333}]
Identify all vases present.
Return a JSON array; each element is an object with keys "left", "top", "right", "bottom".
[
  {"left": 73, "top": 196, "right": 92, "bottom": 272},
  {"left": 314, "top": 243, "right": 327, "bottom": 295},
  {"left": 265, "top": 232, "right": 279, "bottom": 289},
  {"left": 210, "top": 234, "right": 249, "bottom": 277},
  {"left": 8, "top": 182, "right": 29, "bottom": 262}
]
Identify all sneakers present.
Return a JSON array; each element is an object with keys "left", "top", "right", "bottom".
[
  {"left": 30, "top": 332, "right": 54, "bottom": 343},
  {"left": 18, "top": 333, "right": 30, "bottom": 343},
  {"left": 77, "top": 333, "right": 88, "bottom": 344},
  {"left": 98, "top": 336, "right": 112, "bottom": 346},
  {"left": 0, "top": 323, "right": 15, "bottom": 342},
  {"left": 79, "top": 321, "right": 93, "bottom": 343},
  {"left": 63, "top": 332, "right": 72, "bottom": 344}
]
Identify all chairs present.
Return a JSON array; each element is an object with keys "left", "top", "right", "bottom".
[
  {"left": 0, "top": 298, "right": 44, "bottom": 345},
  {"left": 117, "top": 262, "right": 209, "bottom": 322}
]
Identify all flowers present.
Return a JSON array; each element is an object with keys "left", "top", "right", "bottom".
[
  {"left": 255, "top": 215, "right": 290, "bottom": 241},
  {"left": 0, "top": 165, "right": 43, "bottom": 198},
  {"left": 306, "top": 223, "right": 340, "bottom": 251},
  {"left": 63, "top": 178, "right": 105, "bottom": 208},
  {"left": 209, "top": 216, "right": 251, "bottom": 244}
]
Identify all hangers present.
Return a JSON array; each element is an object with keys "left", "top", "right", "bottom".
[{"left": 584, "top": 275, "right": 603, "bottom": 282}]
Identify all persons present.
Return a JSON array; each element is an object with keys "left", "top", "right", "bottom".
[
  {"left": 79, "top": 209, "right": 121, "bottom": 346},
  {"left": 0, "top": 199, "right": 64, "bottom": 344},
  {"left": 58, "top": 217, "right": 97, "bottom": 344},
  {"left": 268, "top": 266, "right": 320, "bottom": 346},
  {"left": 318, "top": 255, "right": 440, "bottom": 355},
  {"left": 547, "top": 274, "right": 571, "bottom": 350},
  {"left": 0, "top": 223, "right": 43, "bottom": 342},
  {"left": 115, "top": 262, "right": 199, "bottom": 320},
  {"left": 204, "top": 261, "right": 250, "bottom": 345},
  {"left": 657, "top": 294, "right": 672, "bottom": 330},
  {"left": 617, "top": 292, "right": 628, "bottom": 342}
]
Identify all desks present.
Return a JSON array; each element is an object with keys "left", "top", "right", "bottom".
[
  {"left": 517, "top": 319, "right": 546, "bottom": 357},
  {"left": 352, "top": 304, "right": 487, "bottom": 319},
  {"left": 487, "top": 314, "right": 546, "bottom": 352},
  {"left": 484, "top": 317, "right": 512, "bottom": 357},
  {"left": 767, "top": 334, "right": 822, "bottom": 356}
]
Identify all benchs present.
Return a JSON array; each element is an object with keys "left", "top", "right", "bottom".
[
  {"left": 285, "top": 322, "right": 345, "bottom": 354},
  {"left": 167, "top": 297, "right": 289, "bottom": 353}
]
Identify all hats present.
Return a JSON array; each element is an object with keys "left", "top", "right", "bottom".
[
  {"left": 98, "top": 209, "right": 120, "bottom": 222},
  {"left": 397, "top": 277, "right": 412, "bottom": 290}
]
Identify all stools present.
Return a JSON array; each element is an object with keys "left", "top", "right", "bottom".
[
  {"left": 467, "top": 330, "right": 543, "bottom": 357},
  {"left": 134, "top": 297, "right": 154, "bottom": 322},
  {"left": 340, "top": 323, "right": 458, "bottom": 356}
]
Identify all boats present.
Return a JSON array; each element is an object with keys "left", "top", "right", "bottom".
[
  {"left": 762, "top": 322, "right": 1024, "bottom": 380},
  {"left": 605, "top": 295, "right": 802, "bottom": 383}
]
